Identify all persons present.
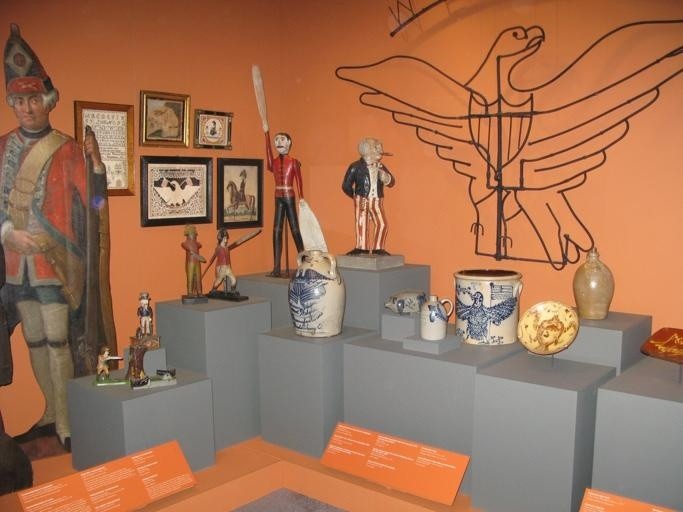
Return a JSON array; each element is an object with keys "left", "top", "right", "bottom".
[
  {"left": 235, "top": 169, "right": 248, "bottom": 209},
  {"left": 342, "top": 136, "right": 396, "bottom": 256},
  {"left": 251, "top": 64, "right": 328, "bottom": 278},
  {"left": 0, "top": 21, "right": 121, "bottom": 452},
  {"left": 136, "top": 291, "right": 154, "bottom": 336},
  {"left": 94, "top": 346, "right": 123, "bottom": 383},
  {"left": 200, "top": 227, "right": 263, "bottom": 302},
  {"left": 181, "top": 225, "right": 207, "bottom": 303}
]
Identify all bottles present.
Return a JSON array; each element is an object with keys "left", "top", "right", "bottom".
[
  {"left": 572, "top": 247, "right": 616, "bottom": 321},
  {"left": 286, "top": 249, "right": 348, "bottom": 338},
  {"left": 419, "top": 294, "right": 454, "bottom": 342}
]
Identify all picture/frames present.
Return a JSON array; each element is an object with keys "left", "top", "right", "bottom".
[
  {"left": 137, "top": 89, "right": 192, "bottom": 149},
  {"left": 71, "top": 99, "right": 137, "bottom": 198},
  {"left": 193, "top": 107, "right": 233, "bottom": 150},
  {"left": 139, "top": 154, "right": 214, "bottom": 227},
  {"left": 215, "top": 157, "right": 264, "bottom": 232}
]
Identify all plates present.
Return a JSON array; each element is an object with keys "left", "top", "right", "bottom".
[
  {"left": 641, "top": 326, "right": 683, "bottom": 365},
  {"left": 516, "top": 300, "right": 580, "bottom": 356}
]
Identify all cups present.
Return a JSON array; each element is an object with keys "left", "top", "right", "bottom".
[{"left": 453, "top": 268, "right": 522, "bottom": 347}]
